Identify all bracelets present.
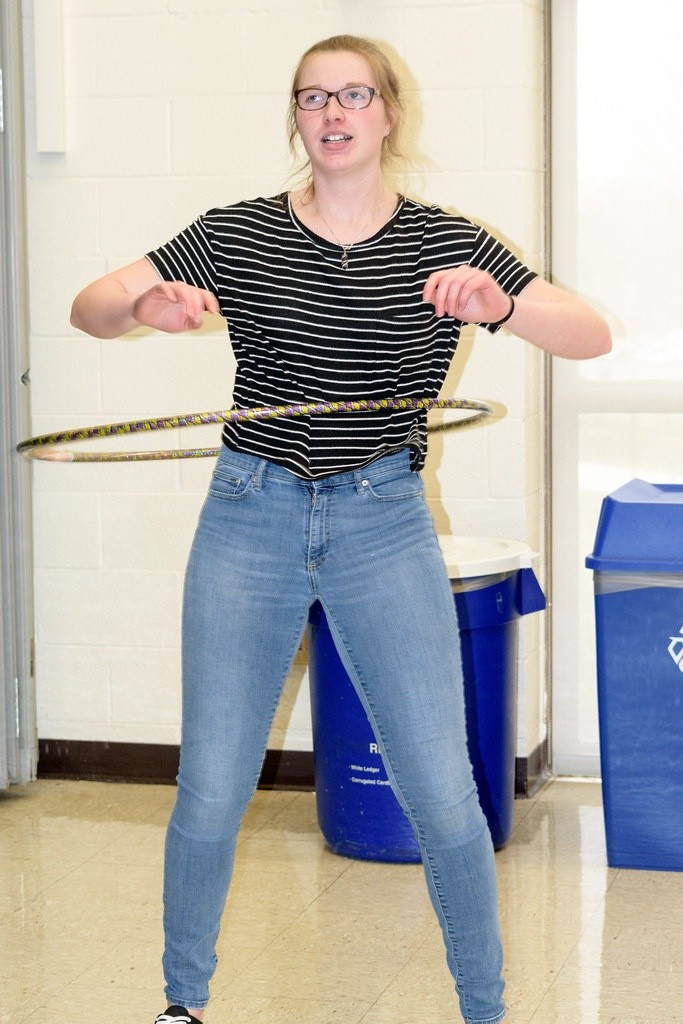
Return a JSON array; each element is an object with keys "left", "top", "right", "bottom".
[{"left": 494, "top": 296, "right": 515, "bottom": 326}]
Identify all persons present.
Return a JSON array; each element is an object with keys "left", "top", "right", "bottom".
[{"left": 68, "top": 34, "right": 610, "bottom": 1024}]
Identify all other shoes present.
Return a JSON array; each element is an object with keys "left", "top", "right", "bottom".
[{"left": 154, "top": 1002, "right": 201, "bottom": 1022}]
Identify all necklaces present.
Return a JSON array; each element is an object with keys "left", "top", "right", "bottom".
[{"left": 309, "top": 187, "right": 382, "bottom": 271}]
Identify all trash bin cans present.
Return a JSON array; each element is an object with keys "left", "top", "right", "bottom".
[
  {"left": 586, "top": 478, "right": 683, "bottom": 870},
  {"left": 308, "top": 535, "right": 546, "bottom": 866}
]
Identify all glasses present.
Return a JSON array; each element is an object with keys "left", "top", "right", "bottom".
[{"left": 294, "top": 83, "right": 383, "bottom": 113}]
